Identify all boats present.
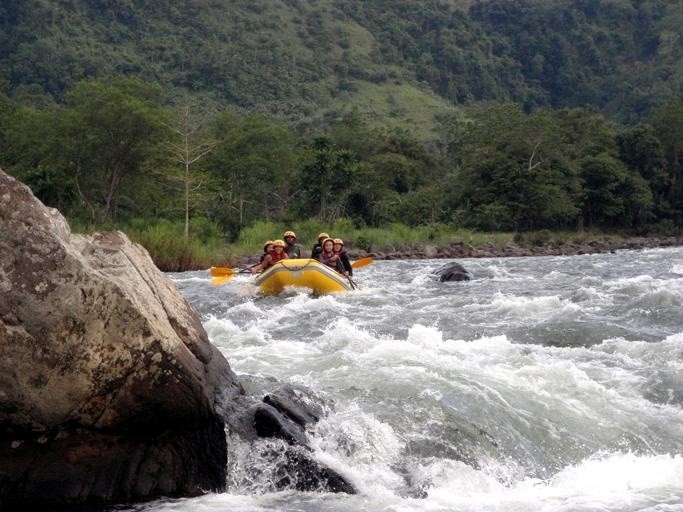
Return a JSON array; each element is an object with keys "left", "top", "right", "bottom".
[{"left": 243, "top": 259, "right": 353, "bottom": 296}]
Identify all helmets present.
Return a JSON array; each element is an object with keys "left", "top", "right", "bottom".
[
  {"left": 263, "top": 241, "right": 273, "bottom": 252},
  {"left": 333, "top": 239, "right": 344, "bottom": 246},
  {"left": 322, "top": 238, "right": 334, "bottom": 248},
  {"left": 273, "top": 240, "right": 285, "bottom": 249},
  {"left": 318, "top": 233, "right": 329, "bottom": 239},
  {"left": 283, "top": 231, "right": 296, "bottom": 239}
]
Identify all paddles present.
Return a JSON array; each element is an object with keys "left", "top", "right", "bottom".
[
  {"left": 351, "top": 257, "right": 372, "bottom": 269},
  {"left": 211, "top": 263, "right": 262, "bottom": 286}
]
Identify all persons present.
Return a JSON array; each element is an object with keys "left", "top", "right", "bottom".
[
  {"left": 332, "top": 239, "right": 354, "bottom": 281},
  {"left": 282, "top": 231, "right": 301, "bottom": 259},
  {"left": 250, "top": 239, "right": 289, "bottom": 276},
  {"left": 259, "top": 240, "right": 274, "bottom": 269},
  {"left": 310, "top": 232, "right": 330, "bottom": 263},
  {"left": 318, "top": 237, "right": 340, "bottom": 272}
]
